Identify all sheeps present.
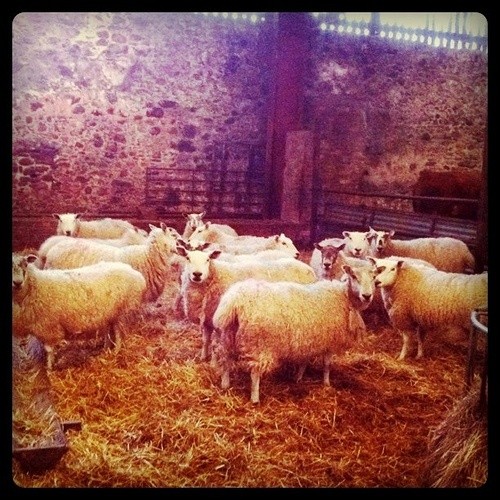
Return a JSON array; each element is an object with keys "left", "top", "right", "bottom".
[
  {"left": 309, "top": 224, "right": 489, "bottom": 363},
  {"left": 210, "top": 266, "right": 386, "bottom": 406},
  {"left": 169, "top": 207, "right": 318, "bottom": 370},
  {"left": 11, "top": 213, "right": 193, "bottom": 378}
]
